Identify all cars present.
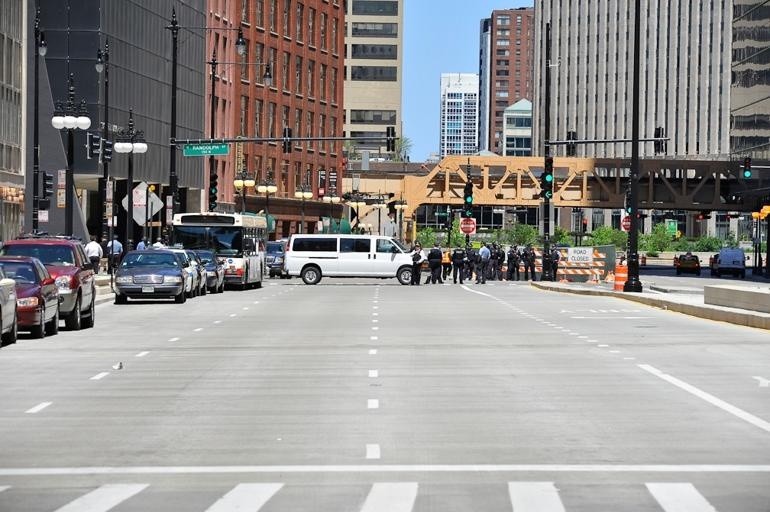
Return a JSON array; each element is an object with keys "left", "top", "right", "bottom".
[
  {"left": 112, "top": 212, "right": 290, "bottom": 304},
  {"left": 0, "top": 263, "right": 18, "bottom": 345},
  {"left": 711, "top": 253, "right": 719, "bottom": 276},
  {"left": 555, "top": 243, "right": 571, "bottom": 253},
  {"left": 676, "top": 252, "right": 702, "bottom": 276},
  {"left": 419, "top": 247, "right": 467, "bottom": 272},
  {"left": 0, "top": 255, "right": 61, "bottom": 339}
]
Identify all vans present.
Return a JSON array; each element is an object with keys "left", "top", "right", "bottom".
[
  {"left": 283, "top": 233, "right": 414, "bottom": 286},
  {"left": 718, "top": 248, "right": 750, "bottom": 279}
]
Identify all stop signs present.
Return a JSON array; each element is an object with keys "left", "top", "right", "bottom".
[
  {"left": 459, "top": 217, "right": 477, "bottom": 235},
  {"left": 621, "top": 214, "right": 631, "bottom": 232}
]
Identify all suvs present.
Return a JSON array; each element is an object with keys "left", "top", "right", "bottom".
[{"left": 0, "top": 234, "right": 97, "bottom": 331}]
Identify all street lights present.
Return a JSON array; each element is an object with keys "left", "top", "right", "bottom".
[
  {"left": 349, "top": 191, "right": 365, "bottom": 234},
  {"left": 372, "top": 190, "right": 386, "bottom": 235},
  {"left": 29, "top": 5, "right": 47, "bottom": 236},
  {"left": 395, "top": 191, "right": 409, "bottom": 244},
  {"left": 94, "top": 36, "right": 110, "bottom": 257},
  {"left": 163, "top": 3, "right": 249, "bottom": 213},
  {"left": 752, "top": 195, "right": 770, "bottom": 279},
  {"left": 233, "top": 166, "right": 256, "bottom": 213},
  {"left": 294, "top": 177, "right": 313, "bottom": 234},
  {"left": 257, "top": 169, "right": 277, "bottom": 217},
  {"left": 52, "top": 74, "right": 91, "bottom": 239},
  {"left": 204, "top": 43, "right": 275, "bottom": 210},
  {"left": 322, "top": 184, "right": 341, "bottom": 234},
  {"left": 113, "top": 107, "right": 149, "bottom": 252}
]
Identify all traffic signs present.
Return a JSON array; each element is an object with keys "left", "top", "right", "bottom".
[
  {"left": 435, "top": 212, "right": 450, "bottom": 216},
  {"left": 183, "top": 142, "right": 229, "bottom": 156}
]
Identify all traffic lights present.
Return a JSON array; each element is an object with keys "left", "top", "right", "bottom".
[
  {"left": 464, "top": 182, "right": 473, "bottom": 217},
  {"left": 583, "top": 217, "right": 587, "bottom": 234},
  {"left": 210, "top": 173, "right": 218, "bottom": 209},
  {"left": 43, "top": 171, "right": 55, "bottom": 198},
  {"left": 744, "top": 156, "right": 752, "bottom": 179},
  {"left": 89, "top": 133, "right": 100, "bottom": 157},
  {"left": 625, "top": 190, "right": 632, "bottom": 213},
  {"left": 637, "top": 213, "right": 648, "bottom": 219},
  {"left": 546, "top": 156, "right": 553, "bottom": 198},
  {"left": 726, "top": 215, "right": 740, "bottom": 218},
  {"left": 696, "top": 215, "right": 711, "bottom": 219},
  {"left": 103, "top": 138, "right": 113, "bottom": 163}
]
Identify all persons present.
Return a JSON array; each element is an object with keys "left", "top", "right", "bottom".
[
  {"left": 410, "top": 241, "right": 562, "bottom": 284},
  {"left": 85, "top": 235, "right": 170, "bottom": 275}
]
